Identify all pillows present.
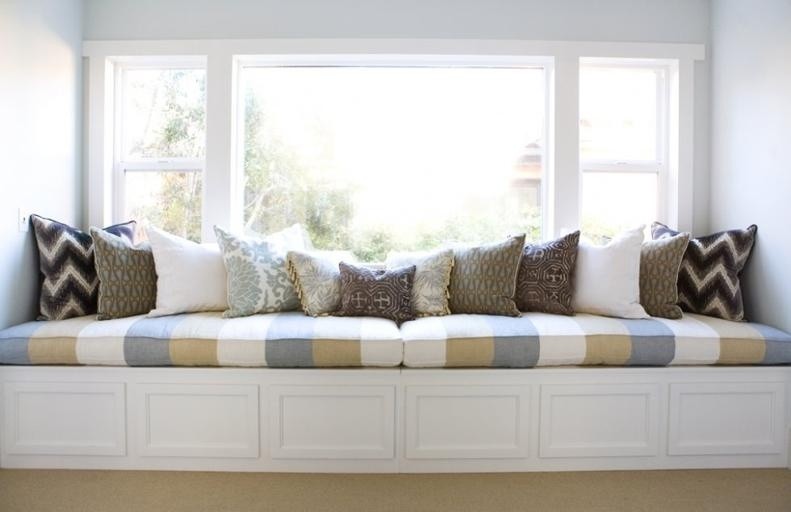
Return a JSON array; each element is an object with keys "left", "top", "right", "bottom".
[
  {"left": 652, "top": 221, "right": 756, "bottom": 322},
  {"left": 142, "top": 222, "right": 227, "bottom": 316},
  {"left": 573, "top": 225, "right": 647, "bottom": 323},
  {"left": 445, "top": 233, "right": 524, "bottom": 317},
  {"left": 282, "top": 247, "right": 358, "bottom": 319},
  {"left": 88, "top": 227, "right": 156, "bottom": 322},
  {"left": 210, "top": 222, "right": 310, "bottom": 319},
  {"left": 30, "top": 213, "right": 137, "bottom": 322},
  {"left": 386, "top": 244, "right": 456, "bottom": 319},
  {"left": 591, "top": 231, "right": 690, "bottom": 320},
  {"left": 333, "top": 259, "right": 418, "bottom": 323},
  {"left": 517, "top": 231, "right": 579, "bottom": 317}
]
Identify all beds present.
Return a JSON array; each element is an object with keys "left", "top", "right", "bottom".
[{"left": 2, "top": 312, "right": 789, "bottom": 474}]
